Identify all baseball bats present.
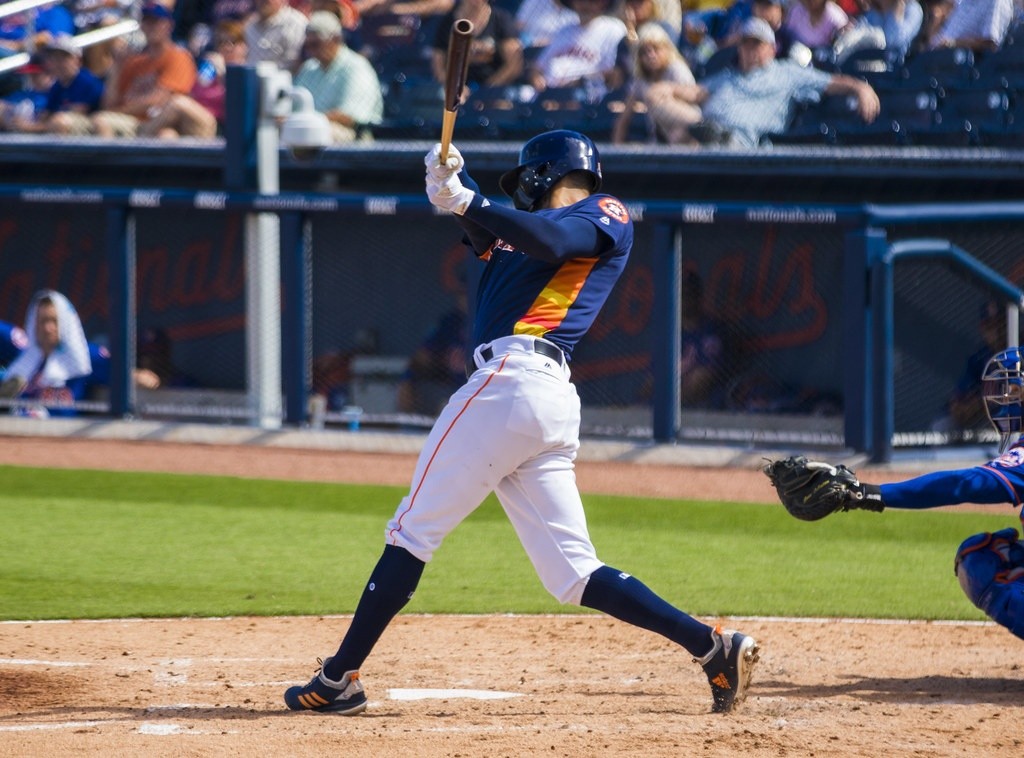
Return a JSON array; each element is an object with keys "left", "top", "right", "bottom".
[{"left": 439, "top": 18, "right": 475, "bottom": 165}]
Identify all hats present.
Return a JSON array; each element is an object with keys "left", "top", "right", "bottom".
[
  {"left": 735, "top": 15, "right": 775, "bottom": 47},
  {"left": 140, "top": 3, "right": 173, "bottom": 19},
  {"left": 304, "top": 10, "right": 341, "bottom": 41},
  {"left": 45, "top": 33, "right": 84, "bottom": 58}
]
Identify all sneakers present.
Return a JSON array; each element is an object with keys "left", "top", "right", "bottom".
[
  {"left": 692, "top": 624, "right": 760, "bottom": 712},
  {"left": 284, "top": 656, "right": 368, "bottom": 715}
]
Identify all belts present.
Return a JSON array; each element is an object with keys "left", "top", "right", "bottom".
[{"left": 465, "top": 339, "right": 562, "bottom": 380}]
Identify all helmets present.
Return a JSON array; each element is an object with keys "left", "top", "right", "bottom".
[
  {"left": 498, "top": 129, "right": 602, "bottom": 211},
  {"left": 982, "top": 346, "right": 1024, "bottom": 434}
]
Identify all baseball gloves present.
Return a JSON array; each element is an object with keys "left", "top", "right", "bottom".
[{"left": 760, "top": 455, "right": 866, "bottom": 521}]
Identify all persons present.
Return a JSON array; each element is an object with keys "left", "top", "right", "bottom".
[
  {"left": 0, "top": 288, "right": 188, "bottom": 418},
  {"left": 285, "top": 130, "right": 760, "bottom": 716},
  {"left": 681, "top": 258, "right": 829, "bottom": 415},
  {"left": 312, "top": 269, "right": 475, "bottom": 409},
  {"left": 0, "top": 0, "right": 1024, "bottom": 146},
  {"left": 954, "top": 297, "right": 1024, "bottom": 430},
  {"left": 762, "top": 348, "right": 1024, "bottom": 641}
]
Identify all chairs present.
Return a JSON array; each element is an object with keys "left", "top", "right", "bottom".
[{"left": 347, "top": 31, "right": 1024, "bottom": 147}]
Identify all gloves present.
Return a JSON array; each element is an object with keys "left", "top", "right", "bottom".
[
  {"left": 425, "top": 141, "right": 464, "bottom": 179},
  {"left": 425, "top": 172, "right": 475, "bottom": 216}
]
